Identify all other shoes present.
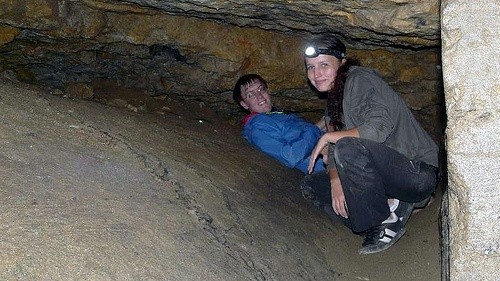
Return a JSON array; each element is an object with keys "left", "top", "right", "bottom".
[{"left": 413, "top": 194, "right": 436, "bottom": 213}]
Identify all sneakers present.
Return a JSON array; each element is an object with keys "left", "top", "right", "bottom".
[
  {"left": 390, "top": 199, "right": 415, "bottom": 226},
  {"left": 358, "top": 212, "right": 408, "bottom": 255}
]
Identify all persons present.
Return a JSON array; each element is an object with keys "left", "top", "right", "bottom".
[
  {"left": 300, "top": 33, "right": 442, "bottom": 256},
  {"left": 233, "top": 74, "right": 435, "bottom": 215}
]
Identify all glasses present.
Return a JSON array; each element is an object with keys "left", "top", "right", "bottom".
[{"left": 242, "top": 85, "right": 266, "bottom": 100}]
